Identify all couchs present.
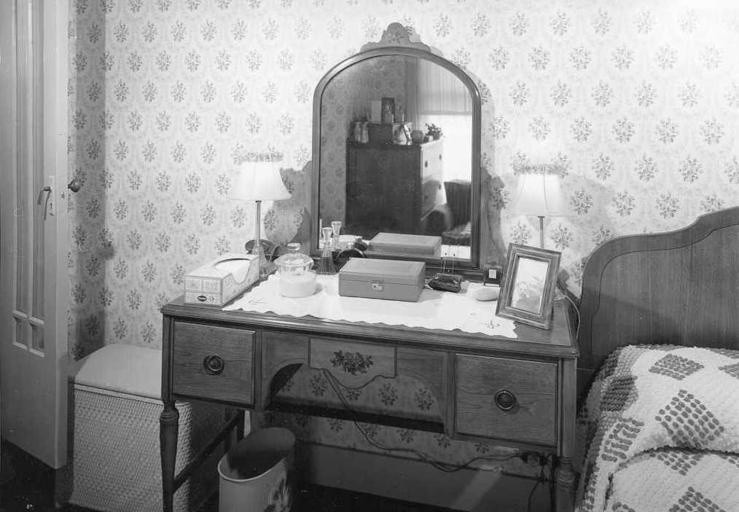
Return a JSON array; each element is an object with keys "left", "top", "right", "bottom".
[{"left": 428, "top": 172, "right": 472, "bottom": 247}]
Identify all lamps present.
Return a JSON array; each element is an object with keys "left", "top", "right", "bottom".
[
  {"left": 509, "top": 170, "right": 568, "bottom": 245},
  {"left": 223, "top": 165, "right": 295, "bottom": 276}
]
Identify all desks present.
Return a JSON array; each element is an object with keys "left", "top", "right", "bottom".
[{"left": 149, "top": 248, "right": 586, "bottom": 512}]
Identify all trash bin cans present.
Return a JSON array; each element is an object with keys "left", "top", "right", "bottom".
[{"left": 217, "top": 427, "right": 296, "bottom": 512}]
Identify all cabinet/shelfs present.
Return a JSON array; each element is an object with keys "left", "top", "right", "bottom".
[{"left": 339, "top": 132, "right": 449, "bottom": 237}]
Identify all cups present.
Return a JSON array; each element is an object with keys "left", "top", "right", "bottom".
[
  {"left": 266, "top": 269, "right": 318, "bottom": 298},
  {"left": 337, "top": 233, "right": 357, "bottom": 248}
]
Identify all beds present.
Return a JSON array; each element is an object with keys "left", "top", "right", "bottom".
[{"left": 563, "top": 206, "right": 739, "bottom": 510}]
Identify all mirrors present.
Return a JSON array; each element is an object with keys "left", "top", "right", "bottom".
[{"left": 311, "top": 46, "right": 481, "bottom": 270}]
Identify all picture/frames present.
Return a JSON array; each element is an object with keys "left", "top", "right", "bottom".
[
  {"left": 493, "top": 245, "right": 566, "bottom": 332},
  {"left": 378, "top": 95, "right": 397, "bottom": 127}
]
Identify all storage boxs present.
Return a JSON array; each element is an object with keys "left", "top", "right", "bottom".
[
  {"left": 365, "top": 231, "right": 443, "bottom": 256},
  {"left": 336, "top": 256, "right": 429, "bottom": 304}
]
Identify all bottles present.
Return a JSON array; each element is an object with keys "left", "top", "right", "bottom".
[
  {"left": 329, "top": 219, "right": 343, "bottom": 250},
  {"left": 316, "top": 227, "right": 336, "bottom": 280},
  {"left": 273, "top": 241, "right": 314, "bottom": 273}
]
